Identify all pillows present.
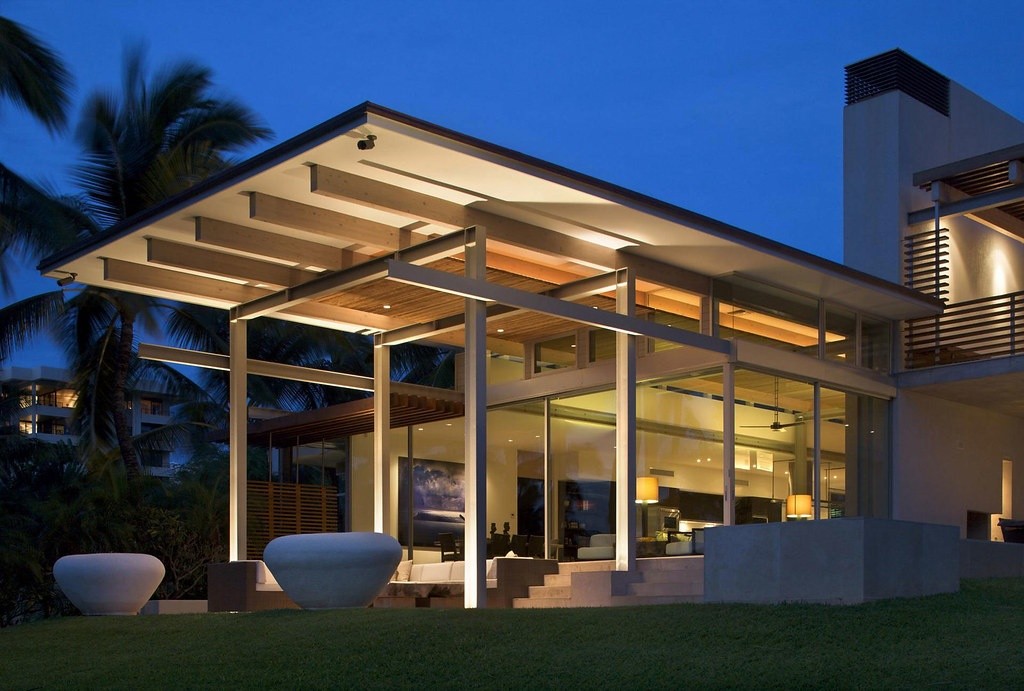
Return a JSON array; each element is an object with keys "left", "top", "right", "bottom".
[
  {"left": 486, "top": 556, "right": 534, "bottom": 579},
  {"left": 391, "top": 560, "right": 412, "bottom": 581}
]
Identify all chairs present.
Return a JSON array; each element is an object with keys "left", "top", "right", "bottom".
[
  {"left": 508, "top": 535, "right": 527, "bottom": 555},
  {"left": 524, "top": 536, "right": 545, "bottom": 557},
  {"left": 439, "top": 532, "right": 463, "bottom": 562},
  {"left": 487, "top": 534, "right": 510, "bottom": 558},
  {"left": 998, "top": 518, "right": 1024, "bottom": 544}
]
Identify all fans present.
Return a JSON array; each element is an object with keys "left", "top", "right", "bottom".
[{"left": 740, "top": 376, "right": 806, "bottom": 432}]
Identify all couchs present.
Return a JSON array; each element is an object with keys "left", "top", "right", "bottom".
[
  {"left": 206, "top": 556, "right": 559, "bottom": 613},
  {"left": 577, "top": 534, "right": 616, "bottom": 560},
  {"left": 666, "top": 528, "right": 704, "bottom": 556}
]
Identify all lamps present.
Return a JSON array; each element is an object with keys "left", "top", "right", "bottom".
[
  {"left": 786, "top": 494, "right": 812, "bottom": 521},
  {"left": 635, "top": 477, "right": 659, "bottom": 536}
]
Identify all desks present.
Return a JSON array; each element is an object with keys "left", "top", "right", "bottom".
[
  {"left": 455, "top": 539, "right": 563, "bottom": 560},
  {"left": 910, "top": 345, "right": 990, "bottom": 367}
]
